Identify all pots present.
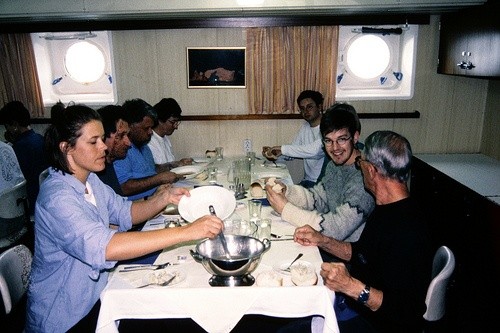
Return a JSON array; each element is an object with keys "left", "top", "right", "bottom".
[{"left": 190, "top": 234, "right": 271, "bottom": 287}]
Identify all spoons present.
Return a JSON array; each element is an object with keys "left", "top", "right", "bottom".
[
  {"left": 192, "top": 160, "right": 201, "bottom": 164},
  {"left": 271, "top": 234, "right": 294, "bottom": 238},
  {"left": 118, "top": 262, "right": 169, "bottom": 272},
  {"left": 273, "top": 161, "right": 280, "bottom": 168}
]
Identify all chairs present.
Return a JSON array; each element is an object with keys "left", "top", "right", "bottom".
[
  {"left": 423, "top": 245, "right": 455, "bottom": 333},
  {"left": 0, "top": 169, "right": 48, "bottom": 316}
]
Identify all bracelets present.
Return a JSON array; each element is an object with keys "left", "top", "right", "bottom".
[{"left": 356, "top": 283, "right": 371, "bottom": 304}]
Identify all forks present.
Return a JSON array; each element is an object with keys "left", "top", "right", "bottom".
[
  {"left": 280, "top": 253, "right": 303, "bottom": 272},
  {"left": 136, "top": 275, "right": 176, "bottom": 288}
]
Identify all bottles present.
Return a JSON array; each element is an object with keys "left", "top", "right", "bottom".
[{"left": 237, "top": 184, "right": 244, "bottom": 198}]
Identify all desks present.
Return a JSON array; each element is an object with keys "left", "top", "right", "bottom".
[{"left": 95, "top": 155, "right": 339, "bottom": 333}]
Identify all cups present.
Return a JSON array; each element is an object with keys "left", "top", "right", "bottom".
[
  {"left": 233, "top": 200, "right": 271, "bottom": 242},
  {"left": 207, "top": 166, "right": 217, "bottom": 184},
  {"left": 247, "top": 152, "right": 255, "bottom": 166},
  {"left": 215, "top": 147, "right": 223, "bottom": 160},
  {"left": 163, "top": 218, "right": 179, "bottom": 229}
]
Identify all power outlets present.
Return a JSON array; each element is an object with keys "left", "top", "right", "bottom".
[{"left": 243, "top": 139, "right": 251, "bottom": 153}]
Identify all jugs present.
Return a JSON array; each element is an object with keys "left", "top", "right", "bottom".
[{"left": 227, "top": 160, "right": 251, "bottom": 189}]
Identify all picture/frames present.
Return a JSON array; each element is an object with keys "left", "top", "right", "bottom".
[{"left": 186, "top": 46, "right": 247, "bottom": 89}]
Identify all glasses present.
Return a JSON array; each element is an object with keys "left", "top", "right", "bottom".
[
  {"left": 355, "top": 156, "right": 377, "bottom": 173},
  {"left": 322, "top": 135, "right": 352, "bottom": 147},
  {"left": 168, "top": 119, "right": 181, "bottom": 128},
  {"left": 298, "top": 105, "right": 319, "bottom": 112}
]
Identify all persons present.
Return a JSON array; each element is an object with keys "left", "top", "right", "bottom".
[
  {"left": 264, "top": 108, "right": 378, "bottom": 333},
  {"left": 24, "top": 102, "right": 225, "bottom": 333},
  {"left": 262, "top": 89, "right": 365, "bottom": 188},
  {"left": 0, "top": 101, "right": 71, "bottom": 241},
  {"left": 294, "top": 130, "right": 435, "bottom": 333},
  {"left": 93, "top": 95, "right": 194, "bottom": 231}
]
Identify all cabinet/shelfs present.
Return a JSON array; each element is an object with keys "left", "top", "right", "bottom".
[{"left": 437, "top": 8, "right": 500, "bottom": 77}]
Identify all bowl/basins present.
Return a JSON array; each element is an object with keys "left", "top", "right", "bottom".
[{"left": 170, "top": 165, "right": 204, "bottom": 180}]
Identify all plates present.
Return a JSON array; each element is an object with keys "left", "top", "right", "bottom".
[
  {"left": 271, "top": 209, "right": 314, "bottom": 278},
  {"left": 267, "top": 163, "right": 286, "bottom": 169},
  {"left": 177, "top": 186, "right": 236, "bottom": 223},
  {"left": 191, "top": 157, "right": 211, "bottom": 165},
  {"left": 161, "top": 206, "right": 180, "bottom": 217},
  {"left": 148, "top": 266, "right": 188, "bottom": 286},
  {"left": 256, "top": 172, "right": 288, "bottom": 182}
]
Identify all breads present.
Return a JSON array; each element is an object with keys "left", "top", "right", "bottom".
[
  {"left": 266, "top": 177, "right": 284, "bottom": 193},
  {"left": 250, "top": 182, "right": 264, "bottom": 197}
]
